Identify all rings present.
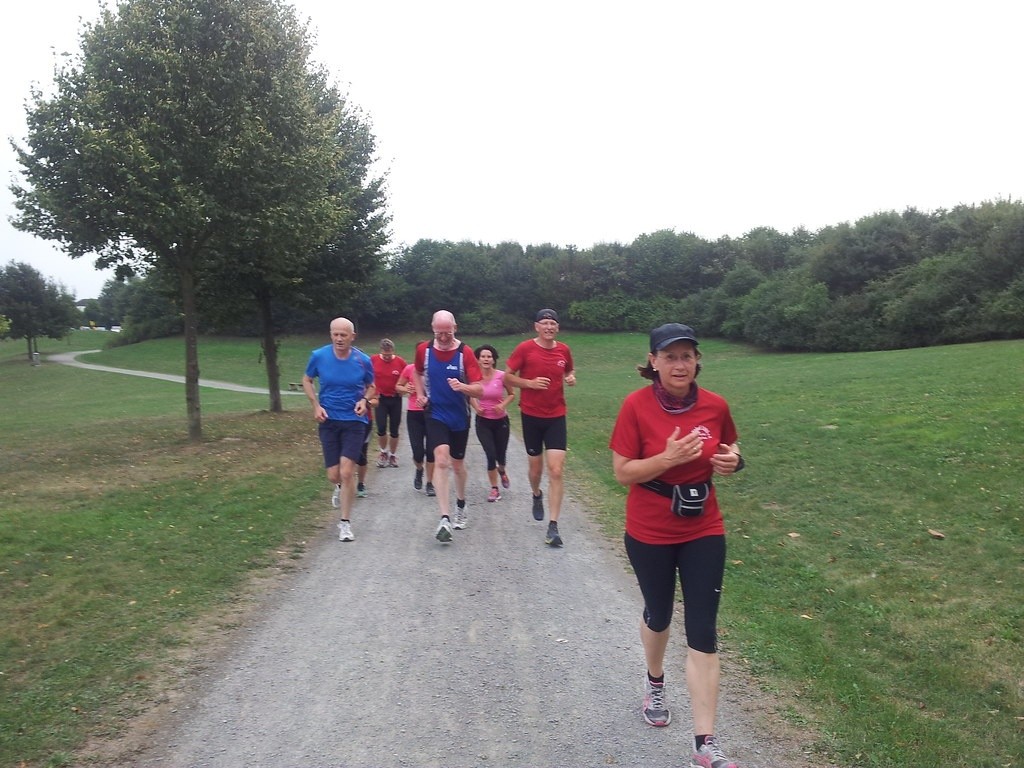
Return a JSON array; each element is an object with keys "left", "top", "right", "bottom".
[{"left": 693, "top": 447, "right": 698, "bottom": 452}]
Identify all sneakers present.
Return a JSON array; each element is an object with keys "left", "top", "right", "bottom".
[
  {"left": 436, "top": 518, "right": 453, "bottom": 542},
  {"left": 332, "top": 485, "right": 341, "bottom": 508},
  {"left": 533, "top": 491, "right": 544, "bottom": 520},
  {"left": 643, "top": 674, "right": 671, "bottom": 726},
  {"left": 546, "top": 530, "right": 563, "bottom": 545},
  {"left": 426, "top": 482, "right": 435, "bottom": 496},
  {"left": 339, "top": 521, "right": 354, "bottom": 541},
  {"left": 453, "top": 508, "right": 470, "bottom": 529},
  {"left": 415, "top": 470, "right": 423, "bottom": 489},
  {"left": 390, "top": 453, "right": 399, "bottom": 467},
  {"left": 376, "top": 450, "right": 387, "bottom": 467},
  {"left": 690, "top": 736, "right": 737, "bottom": 768},
  {"left": 356, "top": 484, "right": 368, "bottom": 497},
  {"left": 497, "top": 468, "right": 509, "bottom": 488},
  {"left": 488, "top": 488, "right": 501, "bottom": 501}
]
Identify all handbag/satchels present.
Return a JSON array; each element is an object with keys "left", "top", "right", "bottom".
[{"left": 671, "top": 484, "right": 709, "bottom": 517}]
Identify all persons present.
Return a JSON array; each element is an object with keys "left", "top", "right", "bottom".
[
  {"left": 502, "top": 310, "right": 576, "bottom": 545},
  {"left": 303, "top": 317, "right": 376, "bottom": 542},
  {"left": 412, "top": 309, "right": 484, "bottom": 543},
  {"left": 610, "top": 322, "right": 746, "bottom": 768},
  {"left": 345, "top": 338, "right": 515, "bottom": 503}
]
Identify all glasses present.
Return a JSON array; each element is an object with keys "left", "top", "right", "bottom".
[
  {"left": 435, "top": 332, "right": 453, "bottom": 338},
  {"left": 539, "top": 322, "right": 559, "bottom": 326},
  {"left": 656, "top": 353, "right": 699, "bottom": 363}
]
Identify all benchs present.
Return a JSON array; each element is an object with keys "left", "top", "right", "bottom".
[{"left": 288, "top": 382, "right": 305, "bottom": 391}]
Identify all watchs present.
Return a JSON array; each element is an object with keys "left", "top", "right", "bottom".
[
  {"left": 734, "top": 451, "right": 745, "bottom": 473},
  {"left": 363, "top": 397, "right": 371, "bottom": 409}
]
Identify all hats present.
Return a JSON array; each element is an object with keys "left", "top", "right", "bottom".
[
  {"left": 650, "top": 323, "right": 699, "bottom": 352},
  {"left": 536, "top": 309, "right": 559, "bottom": 323}
]
